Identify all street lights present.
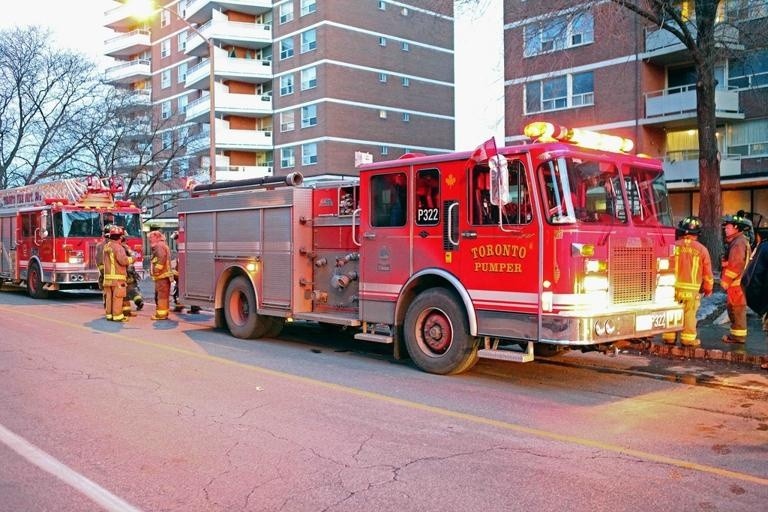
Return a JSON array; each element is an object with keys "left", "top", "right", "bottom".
[{"left": 124, "top": 0, "right": 216, "bottom": 183}]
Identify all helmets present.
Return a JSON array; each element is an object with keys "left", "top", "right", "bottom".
[
  {"left": 675, "top": 215, "right": 705, "bottom": 234},
  {"left": 110, "top": 227, "right": 124, "bottom": 235},
  {"left": 103, "top": 223, "right": 114, "bottom": 236},
  {"left": 145, "top": 230, "right": 167, "bottom": 242},
  {"left": 721, "top": 213, "right": 747, "bottom": 227}
]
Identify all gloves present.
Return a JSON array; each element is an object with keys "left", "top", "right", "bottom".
[
  {"left": 718, "top": 279, "right": 729, "bottom": 289},
  {"left": 703, "top": 288, "right": 712, "bottom": 298}
]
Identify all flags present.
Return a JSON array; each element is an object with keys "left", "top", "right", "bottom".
[{"left": 462, "top": 139, "right": 497, "bottom": 170}]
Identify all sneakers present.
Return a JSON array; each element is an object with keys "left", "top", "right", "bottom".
[
  {"left": 150, "top": 314, "right": 170, "bottom": 321},
  {"left": 662, "top": 338, "right": 702, "bottom": 347},
  {"left": 106, "top": 298, "right": 144, "bottom": 322},
  {"left": 718, "top": 333, "right": 748, "bottom": 344}
]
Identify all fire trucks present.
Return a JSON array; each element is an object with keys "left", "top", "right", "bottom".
[
  {"left": 0, "top": 175, "right": 144, "bottom": 299},
  {"left": 176, "top": 121, "right": 685, "bottom": 376}
]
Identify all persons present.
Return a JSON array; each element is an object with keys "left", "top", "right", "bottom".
[
  {"left": 719, "top": 210, "right": 768, "bottom": 346},
  {"left": 94, "top": 223, "right": 200, "bottom": 322},
  {"left": 662, "top": 215, "right": 714, "bottom": 347}
]
